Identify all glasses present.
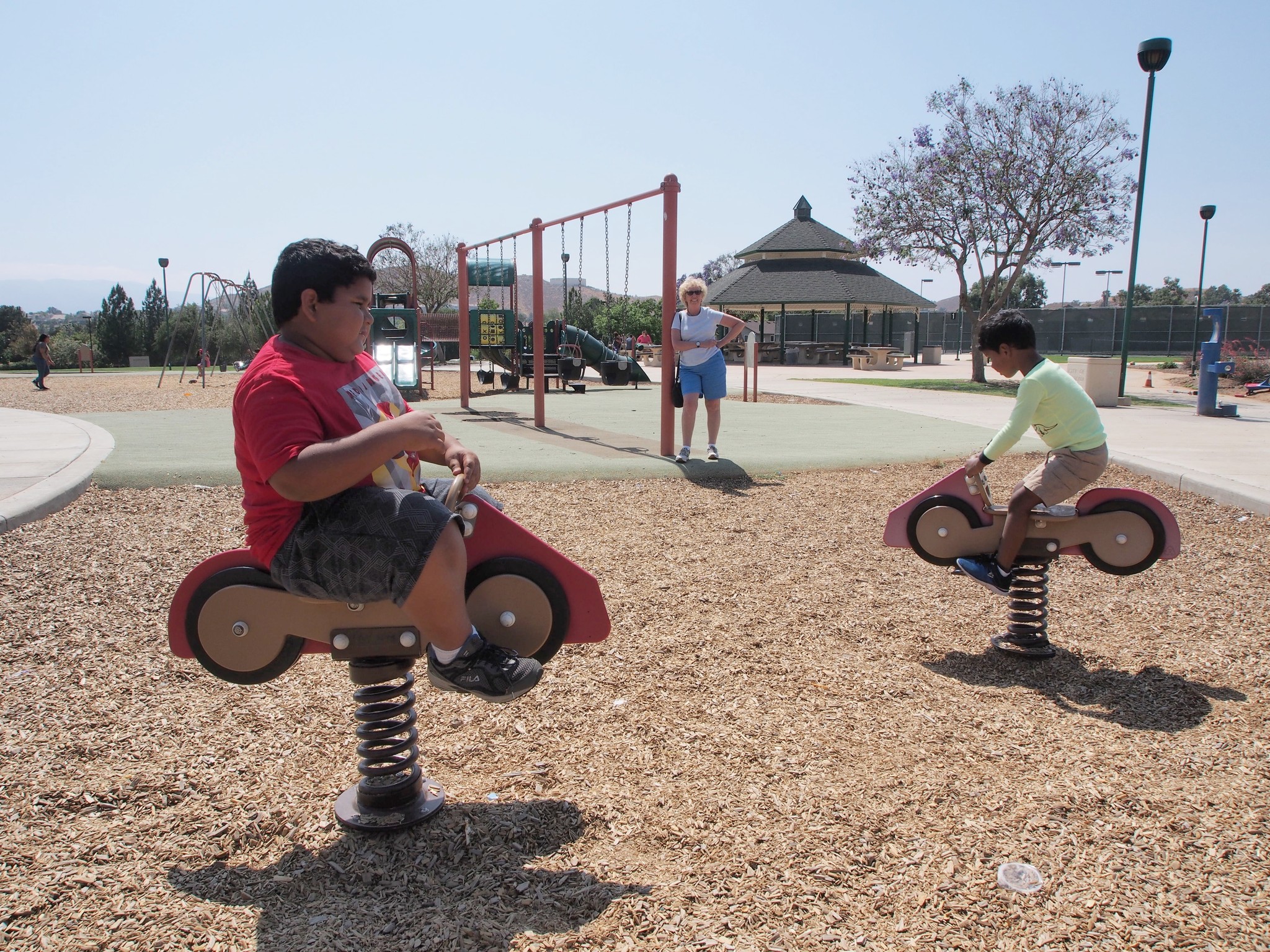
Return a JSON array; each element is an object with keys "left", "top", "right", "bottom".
[{"left": 686, "top": 290, "right": 703, "bottom": 296}]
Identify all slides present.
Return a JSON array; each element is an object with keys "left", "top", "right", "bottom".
[
  {"left": 481, "top": 347, "right": 534, "bottom": 379},
  {"left": 562, "top": 323, "right": 652, "bottom": 383},
  {"left": 372, "top": 340, "right": 419, "bottom": 390},
  {"left": 421, "top": 335, "right": 438, "bottom": 367}
]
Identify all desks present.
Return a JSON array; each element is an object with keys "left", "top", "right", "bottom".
[
  {"left": 642, "top": 345, "right": 662, "bottom": 367},
  {"left": 856, "top": 346, "right": 900, "bottom": 371},
  {"left": 756, "top": 341, "right": 775, "bottom": 363},
  {"left": 784, "top": 343, "right": 829, "bottom": 365},
  {"left": 725, "top": 342, "right": 745, "bottom": 361}
]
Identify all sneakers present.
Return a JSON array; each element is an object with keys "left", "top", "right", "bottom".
[
  {"left": 675, "top": 448, "right": 690, "bottom": 462},
  {"left": 957, "top": 550, "right": 1012, "bottom": 596},
  {"left": 426, "top": 625, "right": 543, "bottom": 704},
  {"left": 707, "top": 445, "right": 719, "bottom": 459}
]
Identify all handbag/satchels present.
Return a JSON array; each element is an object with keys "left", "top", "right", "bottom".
[{"left": 671, "top": 382, "right": 683, "bottom": 408}]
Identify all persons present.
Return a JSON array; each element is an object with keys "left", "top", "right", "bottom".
[
  {"left": 671, "top": 276, "right": 746, "bottom": 462},
  {"left": 612, "top": 330, "right": 653, "bottom": 358},
  {"left": 31, "top": 334, "right": 55, "bottom": 390},
  {"left": 956, "top": 308, "right": 1109, "bottom": 596},
  {"left": 234, "top": 348, "right": 261, "bottom": 372},
  {"left": 195, "top": 349, "right": 210, "bottom": 377},
  {"left": 232, "top": 239, "right": 544, "bottom": 703}
]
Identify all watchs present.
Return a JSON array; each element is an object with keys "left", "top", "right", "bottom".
[{"left": 696, "top": 341, "right": 700, "bottom": 348}]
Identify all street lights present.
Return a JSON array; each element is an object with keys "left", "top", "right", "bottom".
[
  {"left": 1119, "top": 37, "right": 1172, "bottom": 394},
  {"left": 997, "top": 250, "right": 1023, "bottom": 311},
  {"left": 82, "top": 316, "right": 94, "bottom": 368},
  {"left": 158, "top": 258, "right": 172, "bottom": 370},
  {"left": 1095, "top": 271, "right": 1123, "bottom": 307},
  {"left": 921, "top": 279, "right": 933, "bottom": 298},
  {"left": 1051, "top": 262, "right": 1081, "bottom": 307},
  {"left": 1188, "top": 204, "right": 1216, "bottom": 377}
]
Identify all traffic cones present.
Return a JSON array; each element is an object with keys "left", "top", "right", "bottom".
[{"left": 1143, "top": 371, "right": 1155, "bottom": 388}]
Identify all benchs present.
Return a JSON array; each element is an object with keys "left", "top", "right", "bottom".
[
  {"left": 722, "top": 340, "right": 912, "bottom": 372},
  {"left": 608, "top": 343, "right": 680, "bottom": 368},
  {"left": 519, "top": 353, "right": 567, "bottom": 394}
]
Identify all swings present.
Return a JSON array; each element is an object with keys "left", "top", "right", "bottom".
[
  {"left": 499, "top": 235, "right": 521, "bottom": 390},
  {"left": 556, "top": 216, "right": 586, "bottom": 381},
  {"left": 201, "top": 277, "right": 260, "bottom": 372},
  {"left": 601, "top": 202, "right": 633, "bottom": 386},
  {"left": 475, "top": 241, "right": 494, "bottom": 384}
]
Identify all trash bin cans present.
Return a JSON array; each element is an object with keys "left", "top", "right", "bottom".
[
  {"left": 1067, "top": 357, "right": 1122, "bottom": 407},
  {"left": 922, "top": 345, "right": 941, "bottom": 364},
  {"left": 785, "top": 348, "right": 798, "bottom": 365}
]
